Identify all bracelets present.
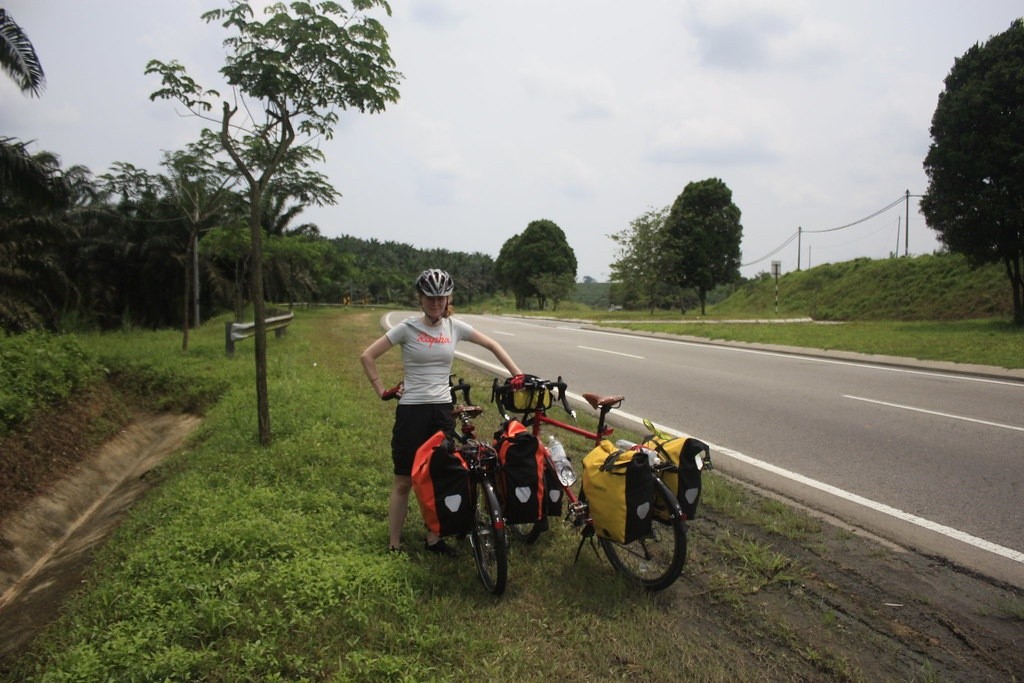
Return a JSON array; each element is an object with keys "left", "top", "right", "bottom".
[{"left": 371, "top": 377, "right": 379, "bottom": 383}]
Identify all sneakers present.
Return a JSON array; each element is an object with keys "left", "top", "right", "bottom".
[{"left": 423, "top": 539, "right": 456, "bottom": 557}]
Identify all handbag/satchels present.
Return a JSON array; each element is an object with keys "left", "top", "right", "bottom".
[
  {"left": 492, "top": 419, "right": 545, "bottom": 524},
  {"left": 641, "top": 433, "right": 713, "bottom": 526},
  {"left": 581, "top": 440, "right": 653, "bottom": 545},
  {"left": 410, "top": 431, "right": 478, "bottom": 537}
]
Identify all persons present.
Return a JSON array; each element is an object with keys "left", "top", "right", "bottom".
[{"left": 359, "top": 268, "right": 524, "bottom": 555}]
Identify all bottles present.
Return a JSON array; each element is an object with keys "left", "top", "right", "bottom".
[
  {"left": 615, "top": 439, "right": 660, "bottom": 466},
  {"left": 548, "top": 436, "right": 577, "bottom": 486}
]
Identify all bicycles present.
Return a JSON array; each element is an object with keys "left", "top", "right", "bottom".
[
  {"left": 492, "top": 375, "right": 715, "bottom": 590},
  {"left": 410, "top": 373, "right": 509, "bottom": 597}
]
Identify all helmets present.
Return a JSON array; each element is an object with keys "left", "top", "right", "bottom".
[{"left": 415, "top": 269, "right": 454, "bottom": 296}]
